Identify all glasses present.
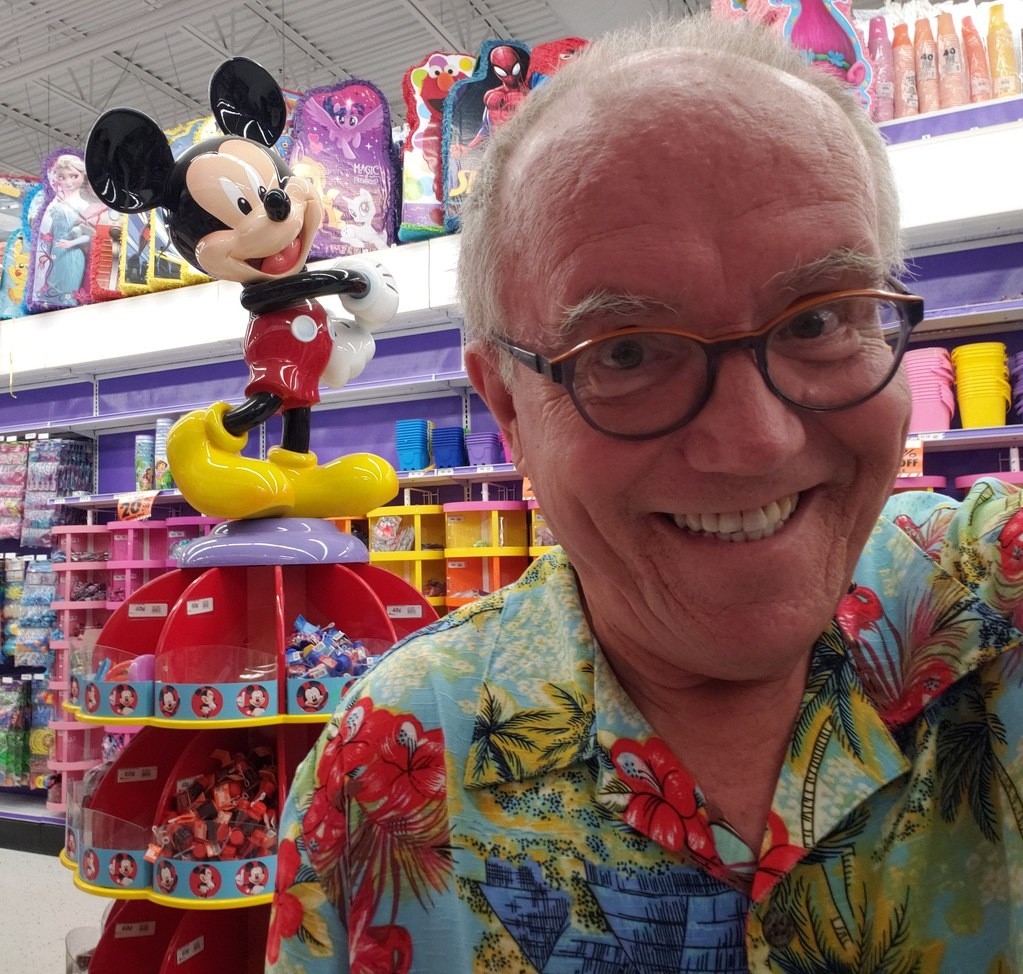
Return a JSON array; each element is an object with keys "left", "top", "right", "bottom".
[{"left": 492, "top": 274, "right": 926, "bottom": 442}]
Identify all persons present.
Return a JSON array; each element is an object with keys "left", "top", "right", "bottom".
[{"left": 263, "top": 15, "right": 1023, "bottom": 974}]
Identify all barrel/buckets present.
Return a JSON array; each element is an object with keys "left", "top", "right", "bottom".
[{"left": 898, "top": 342, "right": 1023, "bottom": 432}]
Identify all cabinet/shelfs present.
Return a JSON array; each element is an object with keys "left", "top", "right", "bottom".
[{"left": 0, "top": 299, "right": 1023, "bottom": 974}]
